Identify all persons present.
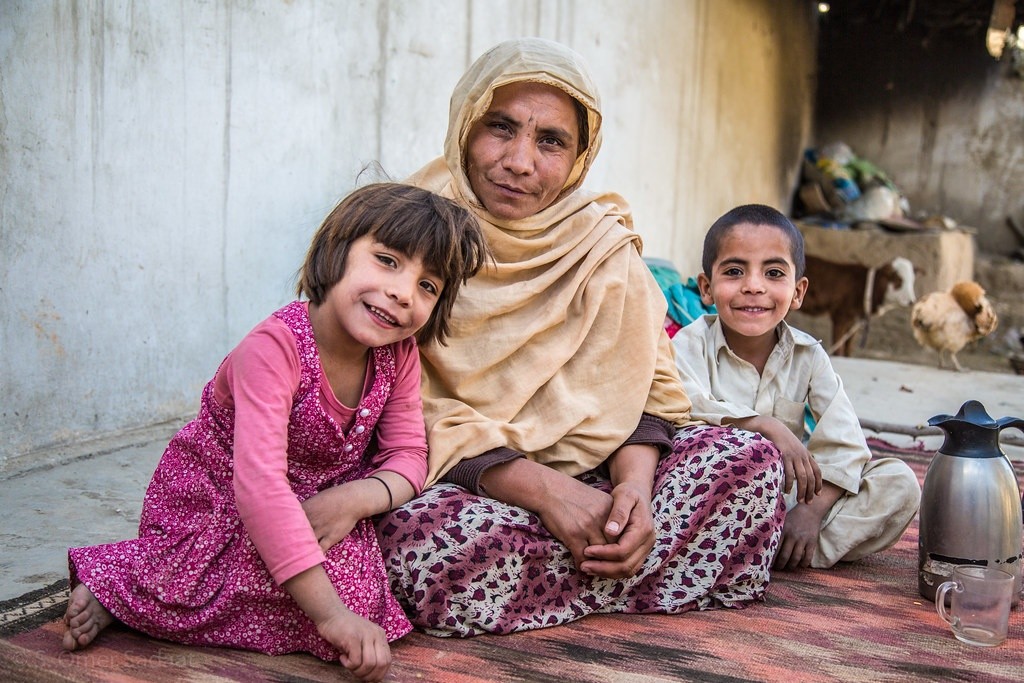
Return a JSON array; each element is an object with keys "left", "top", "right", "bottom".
[
  {"left": 373, "top": 39, "right": 786, "bottom": 638},
  {"left": 671, "top": 203, "right": 921, "bottom": 568},
  {"left": 61, "top": 183, "right": 485, "bottom": 682}
]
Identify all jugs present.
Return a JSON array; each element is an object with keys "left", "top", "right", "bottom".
[{"left": 918, "top": 399, "right": 1024, "bottom": 610}]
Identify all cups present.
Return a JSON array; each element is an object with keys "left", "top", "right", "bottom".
[{"left": 935, "top": 564, "right": 1016, "bottom": 647}]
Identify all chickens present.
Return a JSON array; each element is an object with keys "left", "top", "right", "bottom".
[{"left": 909, "top": 279, "right": 1002, "bottom": 374}]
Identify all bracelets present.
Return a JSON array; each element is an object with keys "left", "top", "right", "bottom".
[{"left": 365, "top": 476, "right": 393, "bottom": 514}]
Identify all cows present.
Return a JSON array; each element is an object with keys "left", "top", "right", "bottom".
[{"left": 795, "top": 251, "right": 919, "bottom": 360}]
